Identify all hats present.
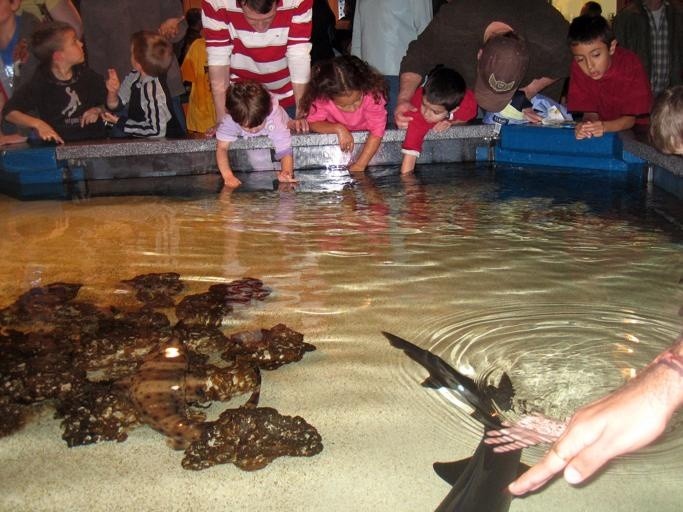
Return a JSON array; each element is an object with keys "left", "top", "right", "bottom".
[{"left": 473, "top": 31, "right": 529, "bottom": 113}]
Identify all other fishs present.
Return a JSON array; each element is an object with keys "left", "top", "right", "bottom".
[{"left": 382, "top": 330, "right": 531, "bottom": 512}]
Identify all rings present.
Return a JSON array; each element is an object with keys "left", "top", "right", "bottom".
[{"left": 347, "top": 145, "right": 349, "bottom": 149}]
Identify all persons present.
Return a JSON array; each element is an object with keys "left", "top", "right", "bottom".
[
  {"left": 400, "top": 175, "right": 481, "bottom": 244},
  {"left": 503, "top": 330, "right": 681, "bottom": 497},
  {"left": 215, "top": 75, "right": 300, "bottom": 188},
  {"left": 217, "top": 188, "right": 296, "bottom": 271},
  {"left": 294, "top": 52, "right": 390, "bottom": 172},
  {"left": 311, "top": 172, "right": 393, "bottom": 263},
  {"left": 397, "top": 65, "right": 480, "bottom": 175},
  {"left": 480, "top": 406, "right": 570, "bottom": 455},
  {"left": 0, "top": 0, "right": 683, "bottom": 157}
]
